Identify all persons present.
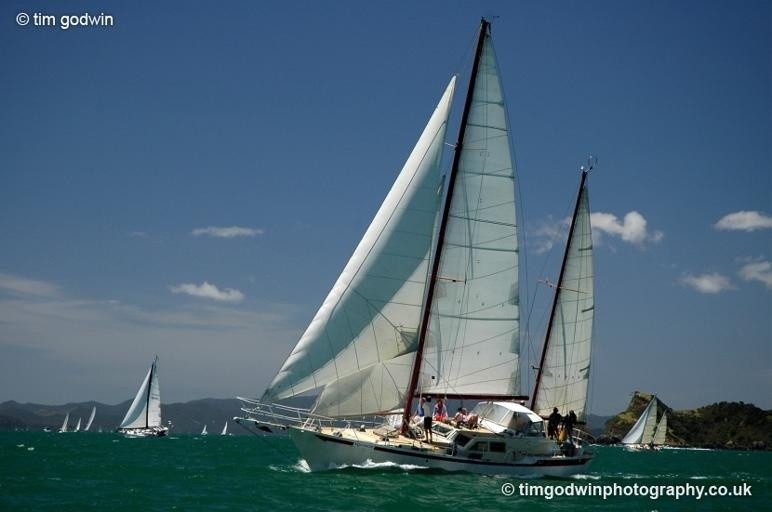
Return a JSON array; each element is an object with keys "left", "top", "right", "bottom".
[
  {"left": 422, "top": 396, "right": 433, "bottom": 444},
  {"left": 561, "top": 409, "right": 586, "bottom": 445},
  {"left": 548, "top": 408, "right": 568, "bottom": 442},
  {"left": 360, "top": 422, "right": 365, "bottom": 432},
  {"left": 432, "top": 398, "right": 448, "bottom": 423},
  {"left": 315, "top": 425, "right": 320, "bottom": 433},
  {"left": 451, "top": 406, "right": 464, "bottom": 429},
  {"left": 414, "top": 398, "right": 424, "bottom": 419}
]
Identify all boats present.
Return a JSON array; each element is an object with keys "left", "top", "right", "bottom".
[{"left": 43, "top": 426, "right": 52, "bottom": 432}]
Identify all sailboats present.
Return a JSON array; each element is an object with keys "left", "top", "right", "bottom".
[
  {"left": 57, "top": 398, "right": 97, "bottom": 433},
  {"left": 236, "top": 17, "right": 595, "bottom": 484},
  {"left": 200, "top": 419, "right": 233, "bottom": 437},
  {"left": 112, "top": 355, "right": 169, "bottom": 438},
  {"left": 621, "top": 389, "right": 669, "bottom": 451}
]
